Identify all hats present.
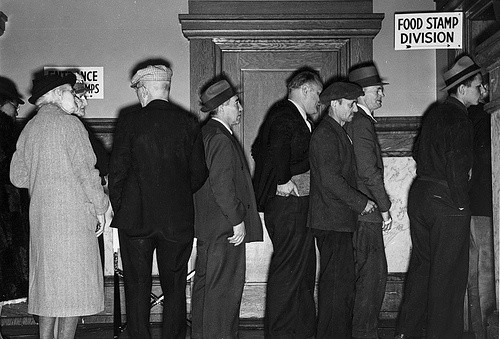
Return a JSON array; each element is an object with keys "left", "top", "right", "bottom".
[
  {"left": 74, "top": 72, "right": 87, "bottom": 92},
  {"left": 0, "top": 76, "right": 25, "bottom": 105},
  {"left": 318, "top": 81, "right": 365, "bottom": 104},
  {"left": 200, "top": 80, "right": 243, "bottom": 112},
  {"left": 440, "top": 56, "right": 485, "bottom": 92},
  {"left": 349, "top": 66, "right": 390, "bottom": 88},
  {"left": 129, "top": 64, "right": 173, "bottom": 88},
  {"left": 28, "top": 68, "right": 77, "bottom": 105}
]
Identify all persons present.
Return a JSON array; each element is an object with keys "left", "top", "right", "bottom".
[{"left": 0, "top": 52, "right": 499, "bottom": 339}]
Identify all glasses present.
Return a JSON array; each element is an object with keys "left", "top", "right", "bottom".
[
  {"left": 64, "top": 89, "right": 76, "bottom": 97},
  {"left": 9, "top": 102, "right": 20, "bottom": 111}
]
